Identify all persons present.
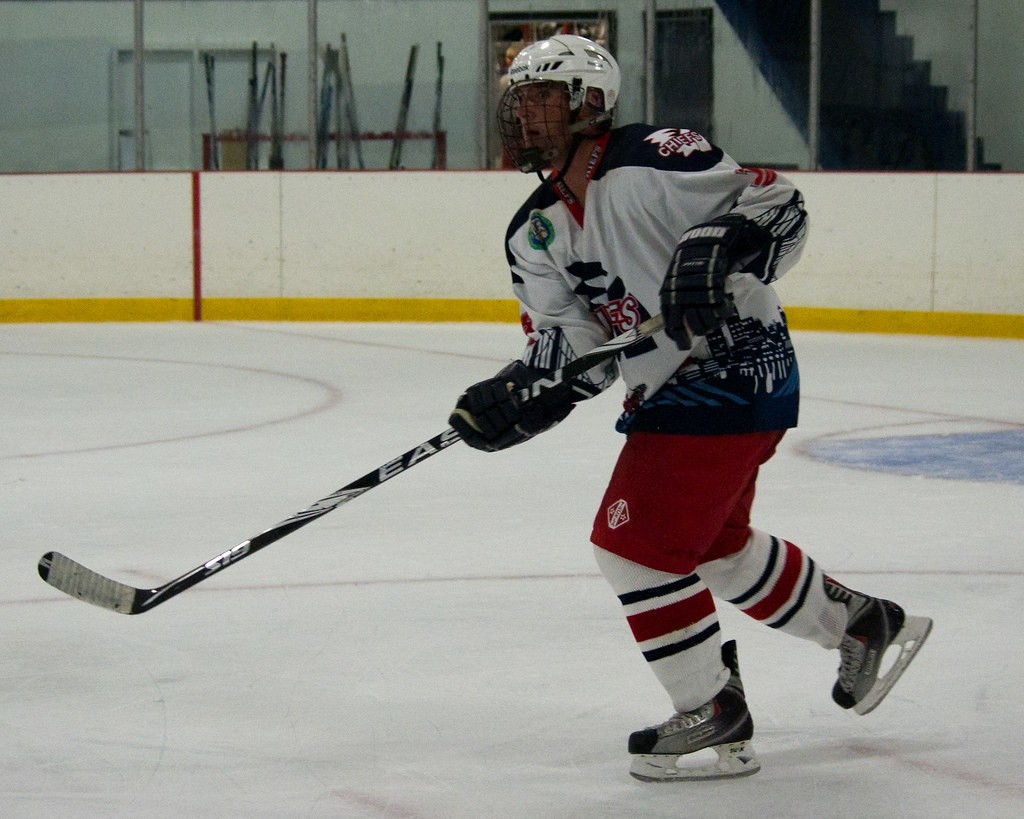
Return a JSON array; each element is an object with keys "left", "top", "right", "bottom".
[{"left": 447, "top": 30, "right": 935, "bottom": 784}]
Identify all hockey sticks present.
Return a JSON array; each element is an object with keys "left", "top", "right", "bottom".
[
  {"left": 199, "top": 31, "right": 449, "bottom": 168},
  {"left": 37, "top": 273, "right": 784, "bottom": 614}
]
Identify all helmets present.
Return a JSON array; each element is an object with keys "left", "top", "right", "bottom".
[{"left": 508, "top": 34, "right": 624, "bottom": 118}]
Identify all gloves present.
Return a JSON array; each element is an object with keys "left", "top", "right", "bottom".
[
  {"left": 658, "top": 213, "right": 769, "bottom": 352},
  {"left": 447, "top": 359, "right": 577, "bottom": 452}
]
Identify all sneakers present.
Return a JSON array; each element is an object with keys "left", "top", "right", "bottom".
[
  {"left": 627, "top": 637, "right": 761, "bottom": 784},
  {"left": 820, "top": 578, "right": 934, "bottom": 717}
]
